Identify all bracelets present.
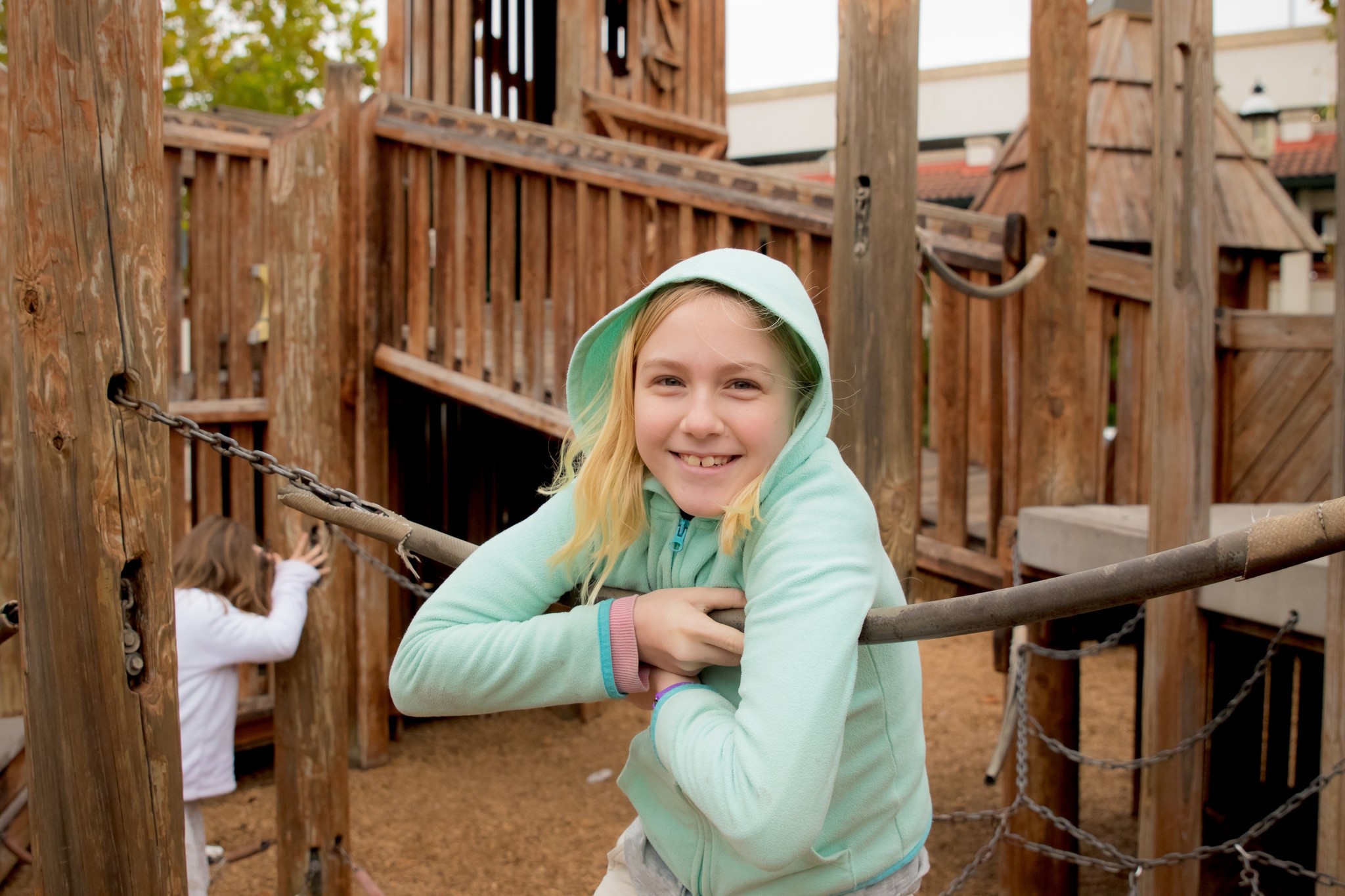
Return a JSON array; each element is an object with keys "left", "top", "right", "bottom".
[{"left": 651, "top": 680, "right": 697, "bottom": 710}]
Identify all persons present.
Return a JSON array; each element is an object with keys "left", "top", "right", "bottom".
[
  {"left": 174, "top": 514, "right": 336, "bottom": 895},
  {"left": 391, "top": 246, "right": 933, "bottom": 896}
]
